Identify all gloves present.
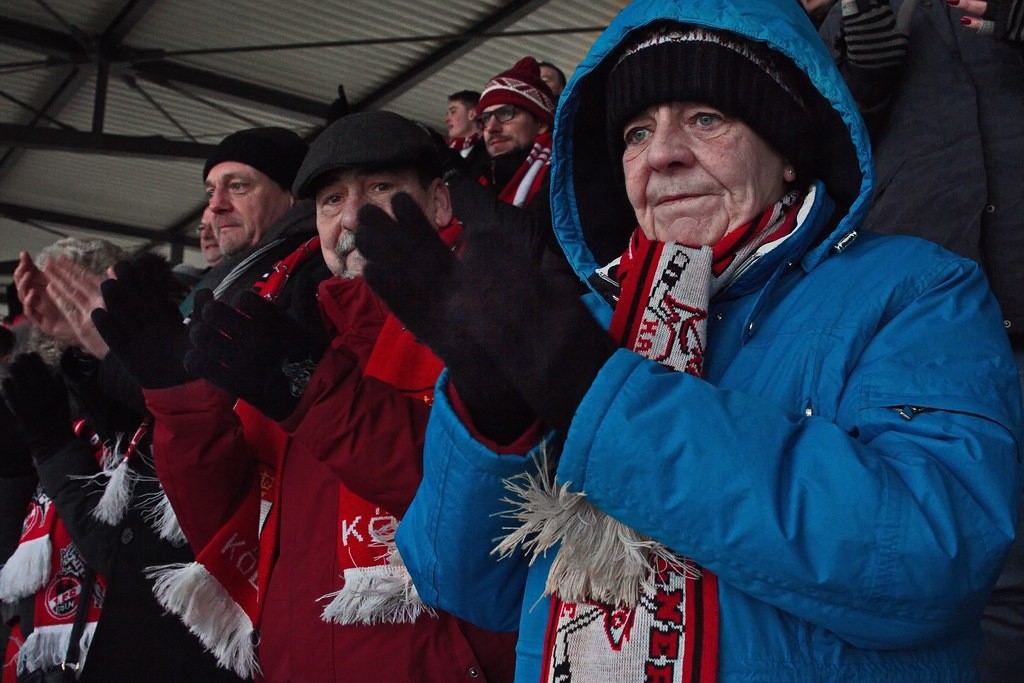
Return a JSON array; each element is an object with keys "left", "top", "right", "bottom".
[
  {"left": 326, "top": 82, "right": 351, "bottom": 127},
  {"left": 353, "top": 191, "right": 537, "bottom": 444},
  {"left": 0, "top": 351, "right": 80, "bottom": 466},
  {"left": 842, "top": 0, "right": 918, "bottom": 108},
  {"left": 182, "top": 271, "right": 333, "bottom": 423},
  {"left": 91, "top": 260, "right": 214, "bottom": 390},
  {"left": 410, "top": 117, "right": 448, "bottom": 166},
  {"left": 445, "top": 199, "right": 617, "bottom": 447}
]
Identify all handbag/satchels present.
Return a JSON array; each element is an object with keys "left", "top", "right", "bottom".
[{"left": 16, "top": 659, "right": 80, "bottom": 683}]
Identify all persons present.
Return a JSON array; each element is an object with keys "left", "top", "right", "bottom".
[
  {"left": 354, "top": 0, "right": 1024, "bottom": 683},
  {"left": 0, "top": 126, "right": 333, "bottom": 683},
  {"left": 442, "top": 57, "right": 566, "bottom": 214},
  {"left": 90, "top": 109, "right": 520, "bottom": 683},
  {"left": 801, "top": 1, "right": 1023, "bottom": 683}
]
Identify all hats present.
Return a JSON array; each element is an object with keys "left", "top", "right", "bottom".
[
  {"left": 606, "top": 21, "right": 833, "bottom": 195},
  {"left": 202, "top": 125, "right": 309, "bottom": 182},
  {"left": 475, "top": 55, "right": 559, "bottom": 125},
  {"left": 292, "top": 110, "right": 439, "bottom": 197}
]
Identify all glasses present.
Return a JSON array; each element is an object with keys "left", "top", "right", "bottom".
[
  {"left": 472, "top": 104, "right": 524, "bottom": 133},
  {"left": 192, "top": 223, "right": 215, "bottom": 235}
]
